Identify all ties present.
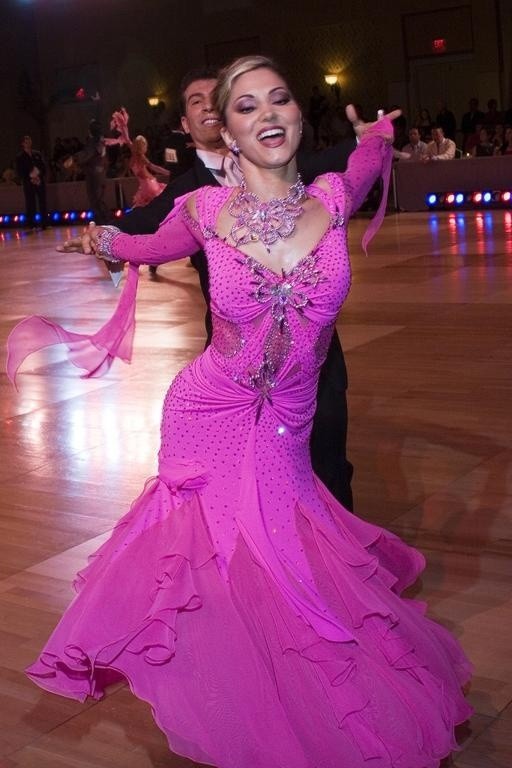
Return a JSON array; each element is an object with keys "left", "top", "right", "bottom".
[{"left": 222, "top": 156, "right": 241, "bottom": 186}]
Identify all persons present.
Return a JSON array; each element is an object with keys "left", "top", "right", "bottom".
[
  {"left": 54, "top": 64, "right": 410, "bottom": 520},
  {"left": 6, "top": 56, "right": 476, "bottom": 768},
  {"left": 388, "top": 97, "right": 511, "bottom": 160},
  {"left": 52, "top": 107, "right": 172, "bottom": 227},
  {"left": 17, "top": 136, "right": 49, "bottom": 231}
]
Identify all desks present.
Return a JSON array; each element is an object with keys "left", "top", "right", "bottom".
[
  {"left": 1, "top": 177, "right": 170, "bottom": 228},
  {"left": 389, "top": 158, "right": 511, "bottom": 211}
]
